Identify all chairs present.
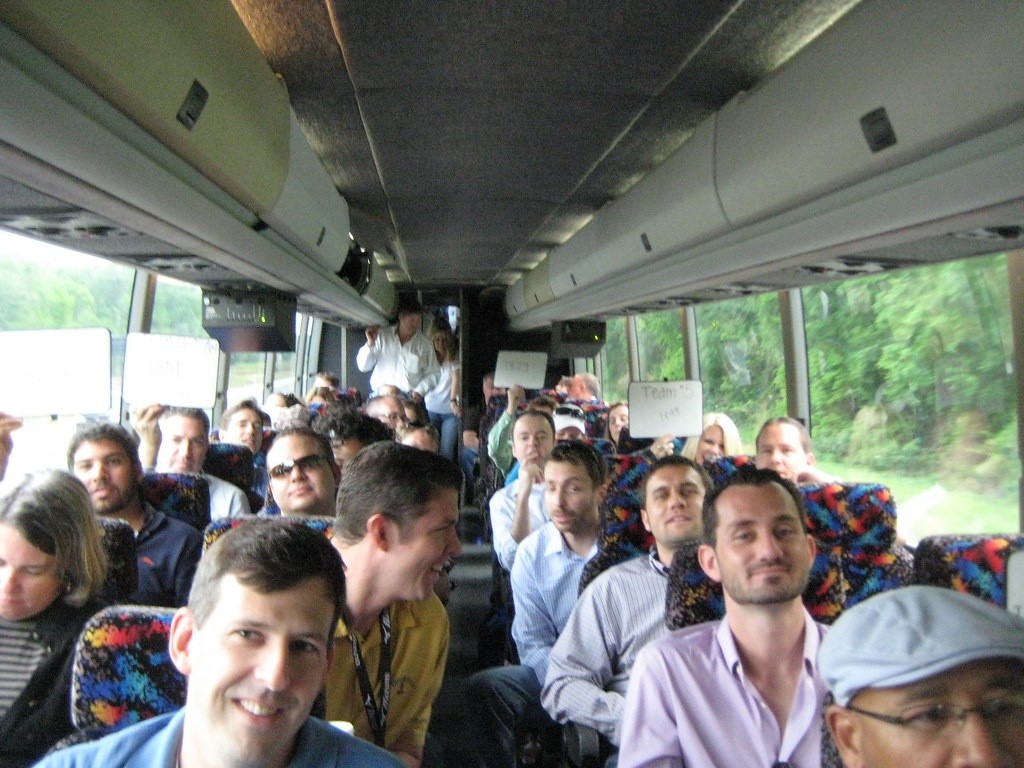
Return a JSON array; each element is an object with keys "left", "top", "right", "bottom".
[
  {"left": 795, "top": 480, "right": 913, "bottom": 609},
  {"left": 575, "top": 461, "right": 657, "bottom": 607},
  {"left": 204, "top": 384, "right": 440, "bottom": 512},
  {"left": 198, "top": 513, "right": 335, "bottom": 565},
  {"left": 659, "top": 529, "right": 847, "bottom": 631},
  {"left": 135, "top": 469, "right": 209, "bottom": 536},
  {"left": 475, "top": 382, "right": 758, "bottom": 487},
  {"left": 912, "top": 533, "right": 1024, "bottom": 615},
  {"left": 43, "top": 599, "right": 187, "bottom": 757},
  {"left": 86, "top": 514, "right": 143, "bottom": 601}
]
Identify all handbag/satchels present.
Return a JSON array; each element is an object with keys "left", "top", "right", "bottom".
[{"left": 338, "top": 241, "right": 374, "bottom": 296}]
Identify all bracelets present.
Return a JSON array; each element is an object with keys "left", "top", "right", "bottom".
[{"left": 450, "top": 398, "right": 459, "bottom": 403}]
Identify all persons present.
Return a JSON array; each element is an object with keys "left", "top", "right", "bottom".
[
  {"left": 540, "top": 457, "right": 713, "bottom": 768},
  {"left": 461, "top": 443, "right": 608, "bottom": 768},
  {"left": 815, "top": 584, "right": 1024, "bottom": 768},
  {"left": 615, "top": 471, "right": 833, "bottom": 768},
  {"left": 327, "top": 440, "right": 463, "bottom": 768},
  {"left": 0, "top": 410, "right": 109, "bottom": 768},
  {"left": 144, "top": 302, "right": 840, "bottom": 522},
  {"left": 475, "top": 413, "right": 556, "bottom": 668},
  {"left": 29, "top": 520, "right": 402, "bottom": 768},
  {"left": 62, "top": 422, "right": 204, "bottom": 609}
]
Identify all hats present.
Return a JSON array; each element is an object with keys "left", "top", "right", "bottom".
[
  {"left": 816, "top": 583, "right": 1024, "bottom": 706},
  {"left": 551, "top": 402, "right": 587, "bottom": 435}
]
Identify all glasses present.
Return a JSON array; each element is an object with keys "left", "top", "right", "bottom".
[
  {"left": 845, "top": 687, "right": 1024, "bottom": 742},
  {"left": 381, "top": 412, "right": 408, "bottom": 424},
  {"left": 554, "top": 437, "right": 604, "bottom": 483},
  {"left": 269, "top": 454, "right": 329, "bottom": 479},
  {"left": 329, "top": 437, "right": 346, "bottom": 448},
  {"left": 554, "top": 407, "right": 587, "bottom": 420}
]
